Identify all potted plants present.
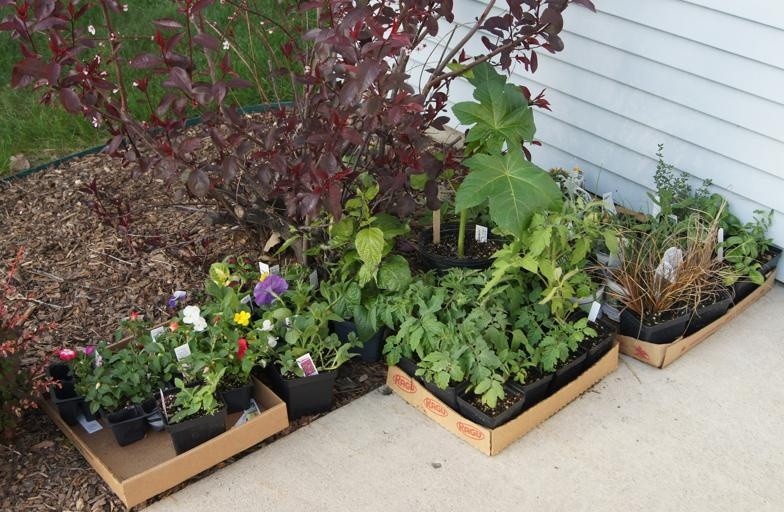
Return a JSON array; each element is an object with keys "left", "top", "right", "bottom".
[
  {"left": 44, "top": 172, "right": 411, "bottom": 454},
  {"left": 363, "top": 62, "right": 782, "bottom": 430}
]
